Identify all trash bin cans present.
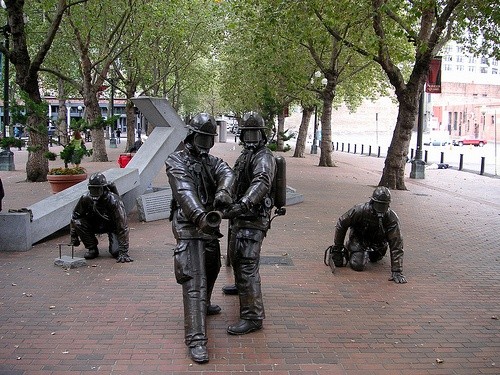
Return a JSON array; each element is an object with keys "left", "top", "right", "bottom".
[
  {"left": 218, "top": 119, "right": 227, "bottom": 144},
  {"left": 118, "top": 153, "right": 133, "bottom": 167}
]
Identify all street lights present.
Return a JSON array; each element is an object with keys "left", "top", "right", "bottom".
[{"left": 311, "top": 71, "right": 327, "bottom": 153}]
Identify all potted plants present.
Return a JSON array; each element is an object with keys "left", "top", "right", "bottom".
[{"left": 1, "top": 82, "right": 121, "bottom": 194}]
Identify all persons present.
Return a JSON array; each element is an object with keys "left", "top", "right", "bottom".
[
  {"left": 221, "top": 111, "right": 277, "bottom": 334},
  {"left": 70, "top": 172, "right": 134, "bottom": 263},
  {"left": 164, "top": 112, "right": 237, "bottom": 363},
  {"left": 116, "top": 128, "right": 122, "bottom": 144},
  {"left": 332, "top": 186, "right": 408, "bottom": 284}
]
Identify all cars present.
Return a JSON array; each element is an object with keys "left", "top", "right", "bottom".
[{"left": 455, "top": 135, "right": 488, "bottom": 147}]
